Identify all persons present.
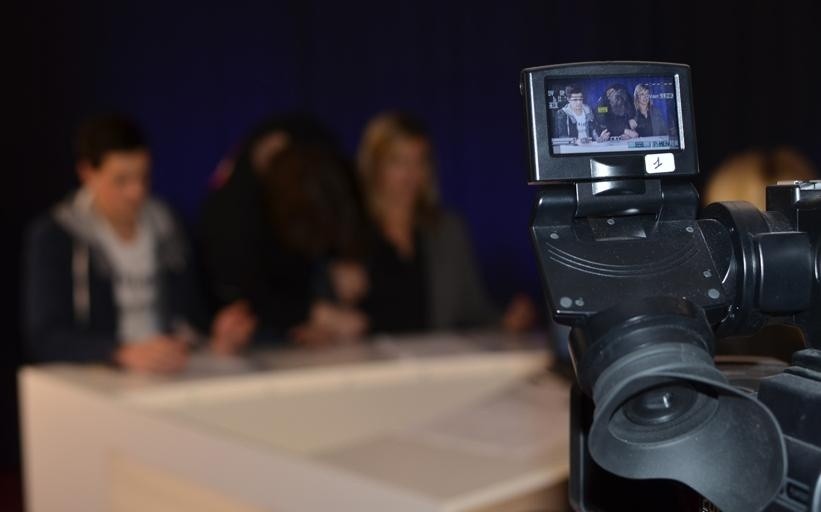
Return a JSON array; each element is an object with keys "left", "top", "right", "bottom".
[
  {"left": 633, "top": 83, "right": 670, "bottom": 138},
  {"left": 595, "top": 84, "right": 640, "bottom": 141},
  {"left": 351, "top": 116, "right": 535, "bottom": 334},
  {"left": 191, "top": 112, "right": 371, "bottom": 348},
  {"left": 555, "top": 85, "right": 612, "bottom": 146},
  {"left": 19, "top": 109, "right": 258, "bottom": 378}
]
